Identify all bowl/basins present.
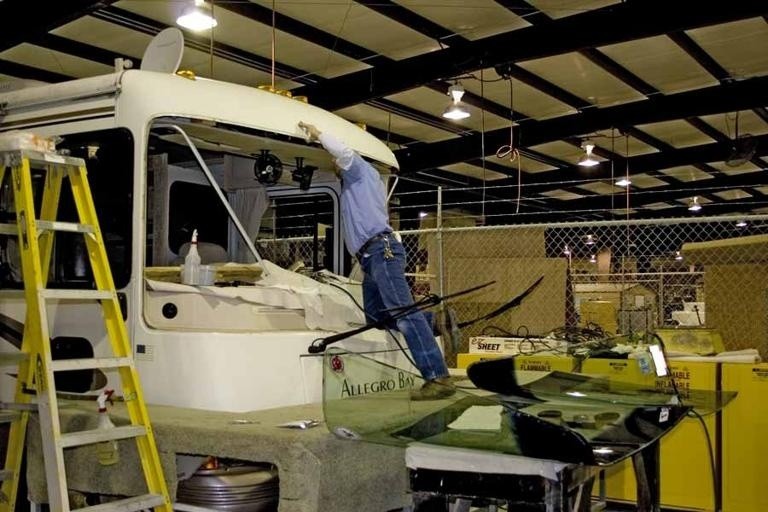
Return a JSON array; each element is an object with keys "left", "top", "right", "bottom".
[{"left": 180, "top": 263, "right": 216, "bottom": 286}]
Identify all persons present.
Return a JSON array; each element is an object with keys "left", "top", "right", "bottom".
[{"left": 299, "top": 121, "right": 457, "bottom": 403}]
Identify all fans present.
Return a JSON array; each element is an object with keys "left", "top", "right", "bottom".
[{"left": 254, "top": 149, "right": 284, "bottom": 185}]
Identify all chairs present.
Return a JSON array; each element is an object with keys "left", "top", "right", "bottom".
[{"left": 179, "top": 242, "right": 230, "bottom": 265}]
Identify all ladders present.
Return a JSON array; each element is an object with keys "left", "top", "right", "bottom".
[{"left": 0, "top": 148, "right": 174, "bottom": 512}]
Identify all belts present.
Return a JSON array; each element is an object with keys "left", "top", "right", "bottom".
[{"left": 355, "top": 231, "right": 390, "bottom": 265}]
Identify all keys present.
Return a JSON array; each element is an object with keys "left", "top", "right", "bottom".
[{"left": 380, "top": 237, "right": 394, "bottom": 259}]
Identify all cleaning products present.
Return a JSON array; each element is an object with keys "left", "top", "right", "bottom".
[
  {"left": 73, "top": 240, "right": 86, "bottom": 277},
  {"left": 181, "top": 229, "right": 201, "bottom": 285},
  {"left": 95, "top": 389, "right": 121, "bottom": 465}
]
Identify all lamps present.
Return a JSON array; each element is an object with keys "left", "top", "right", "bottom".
[
  {"left": 563, "top": 235, "right": 596, "bottom": 262},
  {"left": 175, "top": 1, "right": 218, "bottom": 34},
  {"left": 576, "top": 136, "right": 600, "bottom": 167},
  {"left": 675, "top": 251, "right": 683, "bottom": 260},
  {"left": 442, "top": 78, "right": 471, "bottom": 121},
  {"left": 614, "top": 177, "right": 747, "bottom": 226}
]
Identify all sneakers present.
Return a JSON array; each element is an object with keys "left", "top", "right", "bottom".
[{"left": 409, "top": 380, "right": 458, "bottom": 402}]
